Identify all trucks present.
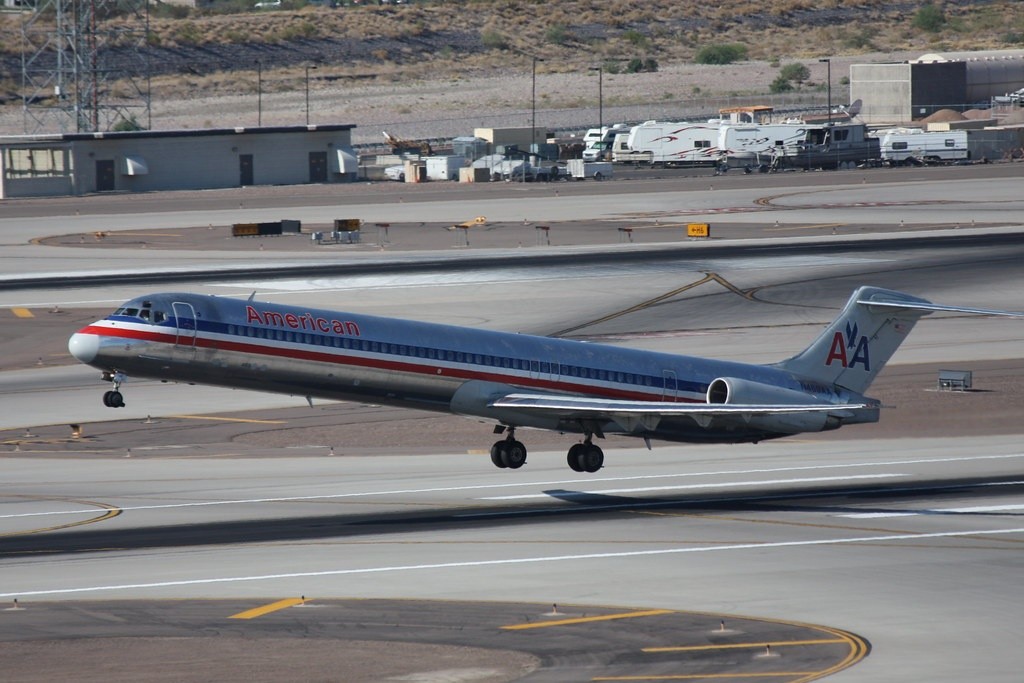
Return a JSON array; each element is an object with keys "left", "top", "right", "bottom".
[{"left": 583, "top": 119, "right": 969, "bottom": 172}]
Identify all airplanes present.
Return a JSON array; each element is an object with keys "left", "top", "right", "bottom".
[{"left": 69, "top": 287, "right": 1024, "bottom": 472}]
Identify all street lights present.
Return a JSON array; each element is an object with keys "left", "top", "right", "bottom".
[
  {"left": 588, "top": 66, "right": 604, "bottom": 162},
  {"left": 531, "top": 58, "right": 545, "bottom": 154},
  {"left": 255, "top": 61, "right": 263, "bottom": 126},
  {"left": 819, "top": 59, "right": 835, "bottom": 126},
  {"left": 305, "top": 64, "right": 317, "bottom": 125}
]
